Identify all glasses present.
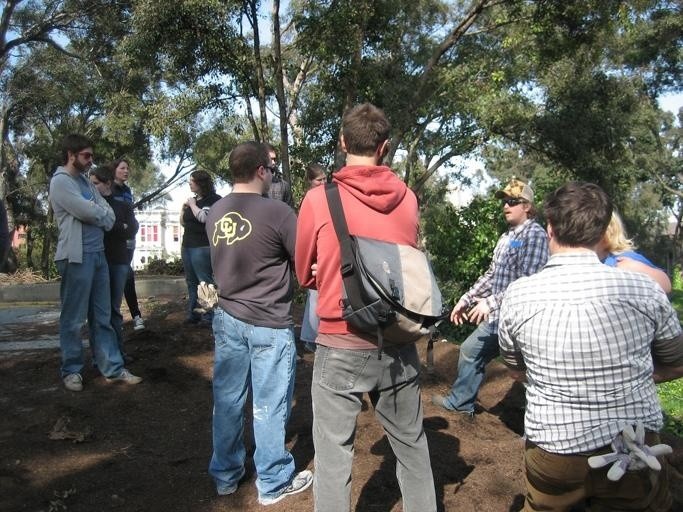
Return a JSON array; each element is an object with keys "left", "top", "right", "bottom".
[
  {"left": 256, "top": 157, "right": 278, "bottom": 175},
  {"left": 75, "top": 151, "right": 91, "bottom": 159},
  {"left": 501, "top": 200, "right": 530, "bottom": 207}
]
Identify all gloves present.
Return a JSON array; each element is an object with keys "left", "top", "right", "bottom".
[
  {"left": 586, "top": 421, "right": 676, "bottom": 486},
  {"left": 192, "top": 280, "right": 222, "bottom": 317}
]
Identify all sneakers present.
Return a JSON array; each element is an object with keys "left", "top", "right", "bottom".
[
  {"left": 103, "top": 366, "right": 143, "bottom": 386},
  {"left": 131, "top": 314, "right": 146, "bottom": 331},
  {"left": 255, "top": 468, "right": 315, "bottom": 507},
  {"left": 215, "top": 480, "right": 238, "bottom": 497},
  {"left": 431, "top": 393, "right": 477, "bottom": 424},
  {"left": 61, "top": 372, "right": 85, "bottom": 392}
]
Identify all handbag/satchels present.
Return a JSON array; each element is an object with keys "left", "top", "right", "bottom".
[{"left": 334, "top": 234, "right": 446, "bottom": 350}]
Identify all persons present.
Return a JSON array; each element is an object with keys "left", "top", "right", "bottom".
[
  {"left": 180, "top": 170, "right": 222, "bottom": 330},
  {"left": 498, "top": 181, "right": 683, "bottom": 512},
  {"left": 205, "top": 140, "right": 313, "bottom": 506},
  {"left": 89, "top": 166, "right": 134, "bottom": 368},
  {"left": 49, "top": 134, "right": 143, "bottom": 392},
  {"left": 294, "top": 102, "right": 437, "bottom": 512},
  {"left": 431, "top": 180, "right": 550, "bottom": 414},
  {"left": 304, "top": 164, "right": 329, "bottom": 188},
  {"left": 113, "top": 158, "right": 145, "bottom": 331},
  {"left": 596, "top": 212, "right": 672, "bottom": 296}
]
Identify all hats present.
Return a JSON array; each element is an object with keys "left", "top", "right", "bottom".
[{"left": 491, "top": 178, "right": 536, "bottom": 203}]
